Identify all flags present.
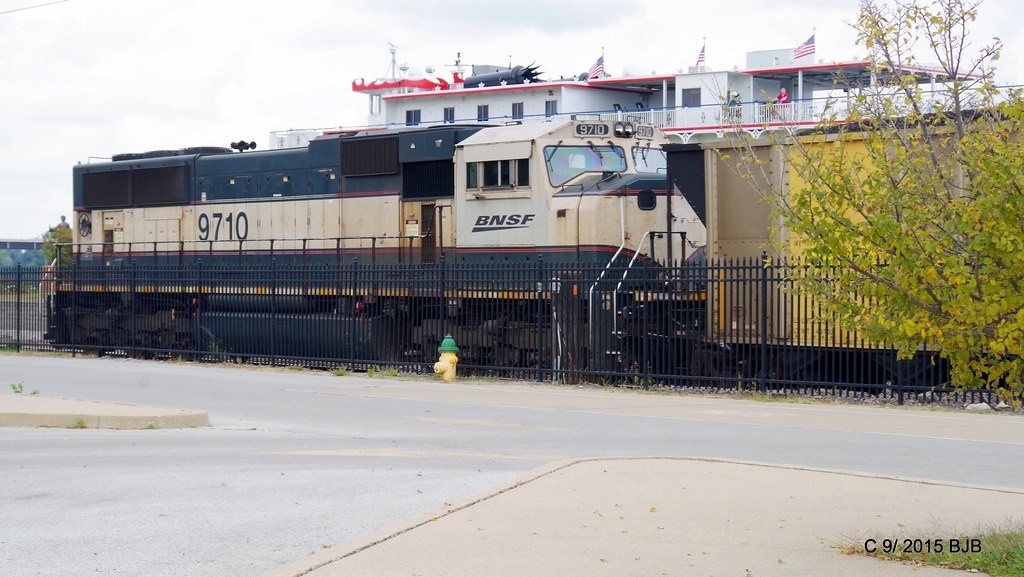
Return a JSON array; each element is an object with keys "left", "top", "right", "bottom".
[
  {"left": 696, "top": 44, "right": 705, "bottom": 65},
  {"left": 793, "top": 34, "right": 815, "bottom": 59},
  {"left": 587, "top": 55, "right": 604, "bottom": 80}
]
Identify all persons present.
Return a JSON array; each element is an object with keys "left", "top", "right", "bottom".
[
  {"left": 769, "top": 87, "right": 790, "bottom": 122},
  {"left": 727, "top": 91, "right": 741, "bottom": 124}
]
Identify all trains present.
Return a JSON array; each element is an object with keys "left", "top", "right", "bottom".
[{"left": 47, "top": 115, "right": 713, "bottom": 376}]
